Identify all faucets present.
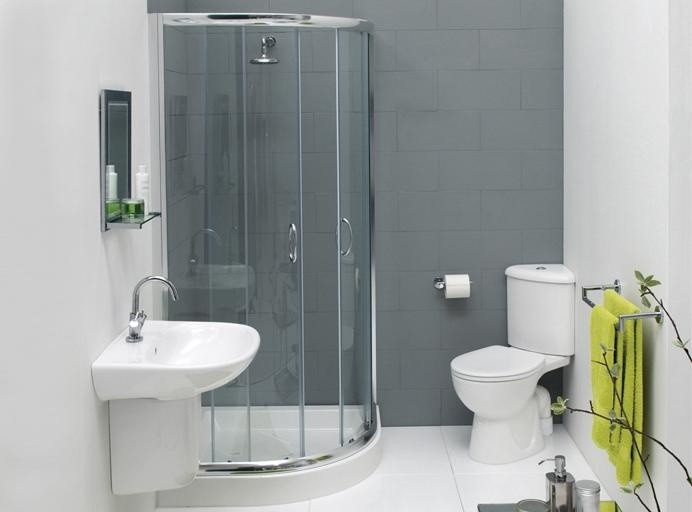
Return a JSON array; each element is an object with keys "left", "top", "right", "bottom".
[
  {"left": 190, "top": 227, "right": 220, "bottom": 272},
  {"left": 130, "top": 275, "right": 180, "bottom": 321}
]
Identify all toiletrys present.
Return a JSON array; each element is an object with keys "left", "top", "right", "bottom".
[{"left": 121, "top": 198, "right": 145, "bottom": 221}]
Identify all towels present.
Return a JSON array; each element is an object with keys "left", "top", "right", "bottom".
[
  {"left": 603, "top": 290, "right": 650, "bottom": 496},
  {"left": 592, "top": 302, "right": 621, "bottom": 449}
]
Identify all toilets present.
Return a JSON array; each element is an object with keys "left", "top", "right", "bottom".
[{"left": 450, "top": 263, "right": 577, "bottom": 464}]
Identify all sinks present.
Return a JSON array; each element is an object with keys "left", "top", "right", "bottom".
[
  {"left": 91, "top": 320, "right": 260, "bottom": 403},
  {"left": 174, "top": 264, "right": 258, "bottom": 311}
]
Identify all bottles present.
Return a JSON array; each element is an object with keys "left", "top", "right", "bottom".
[{"left": 574, "top": 480, "right": 600, "bottom": 512}]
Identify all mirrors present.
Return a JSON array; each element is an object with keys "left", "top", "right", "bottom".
[{"left": 102, "top": 87, "right": 134, "bottom": 232}]
